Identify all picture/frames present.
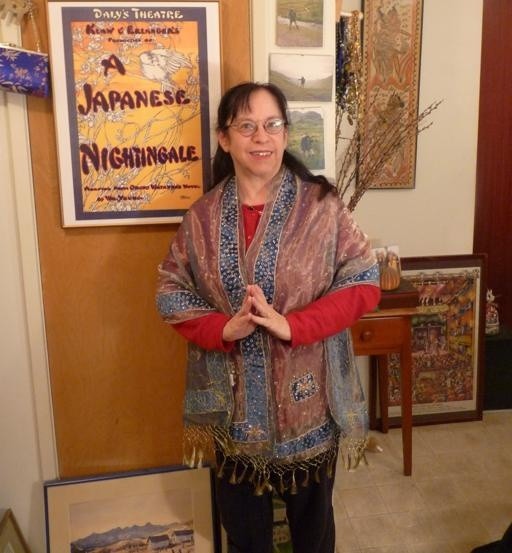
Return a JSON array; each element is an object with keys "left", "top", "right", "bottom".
[
  {"left": 0, "top": 462, "right": 226, "bottom": 553},
  {"left": 372, "top": 253, "right": 489, "bottom": 428},
  {"left": 44, "top": 0, "right": 221, "bottom": 228},
  {"left": 248, "top": 1, "right": 337, "bottom": 184}
]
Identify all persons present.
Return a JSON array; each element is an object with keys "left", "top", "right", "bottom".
[{"left": 153, "top": 79, "right": 386, "bottom": 551}]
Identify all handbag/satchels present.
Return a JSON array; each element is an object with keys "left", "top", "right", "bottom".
[{"left": 0, "top": 43, "right": 50, "bottom": 99}]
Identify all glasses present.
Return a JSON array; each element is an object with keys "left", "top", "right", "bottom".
[{"left": 222, "top": 115, "right": 288, "bottom": 138}]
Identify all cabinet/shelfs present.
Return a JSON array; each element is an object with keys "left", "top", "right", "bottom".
[{"left": 347, "top": 279, "right": 424, "bottom": 477}]
